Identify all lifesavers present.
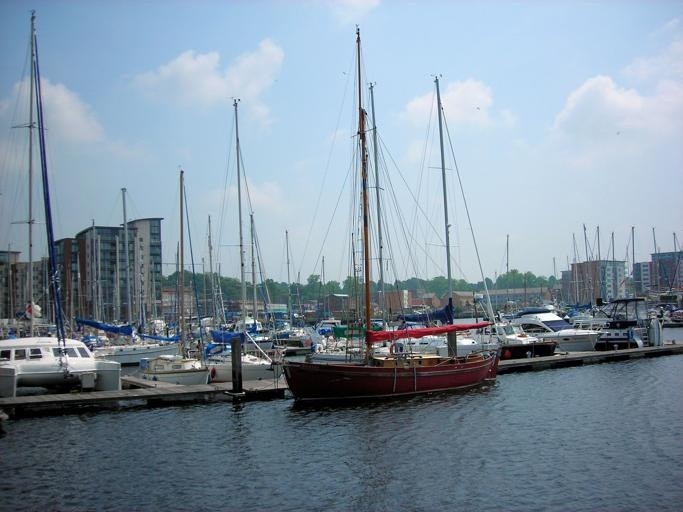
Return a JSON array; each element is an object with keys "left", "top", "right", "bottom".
[
  {"left": 315, "top": 343, "right": 323, "bottom": 353},
  {"left": 390, "top": 343, "right": 403, "bottom": 357}
]
